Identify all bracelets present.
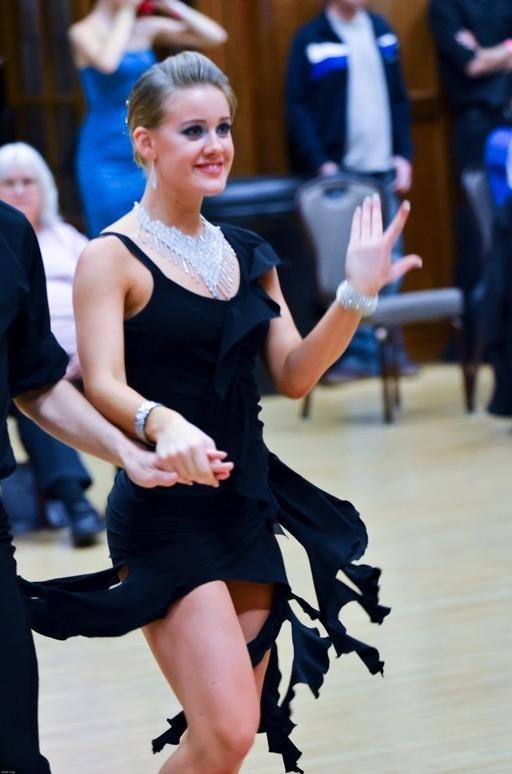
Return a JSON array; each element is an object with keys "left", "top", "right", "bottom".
[
  {"left": 337, "top": 280, "right": 380, "bottom": 316},
  {"left": 134, "top": 399, "right": 161, "bottom": 447}
]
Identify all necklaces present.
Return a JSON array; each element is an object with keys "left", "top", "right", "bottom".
[{"left": 133, "top": 200, "right": 238, "bottom": 300}]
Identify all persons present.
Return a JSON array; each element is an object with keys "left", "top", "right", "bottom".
[
  {"left": 284, "top": 2, "right": 417, "bottom": 382},
  {"left": 426, "top": 2, "right": 512, "bottom": 421},
  {"left": 0, "top": 141, "right": 104, "bottom": 548},
  {"left": 66, "top": 1, "right": 230, "bottom": 239},
  {"left": 0, "top": 199, "right": 235, "bottom": 774},
  {"left": 70, "top": 48, "right": 427, "bottom": 774}
]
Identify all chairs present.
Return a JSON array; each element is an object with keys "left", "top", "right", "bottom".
[{"left": 294, "top": 171, "right": 481, "bottom": 432}]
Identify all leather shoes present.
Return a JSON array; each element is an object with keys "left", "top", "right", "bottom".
[{"left": 67, "top": 498, "right": 104, "bottom": 543}]
